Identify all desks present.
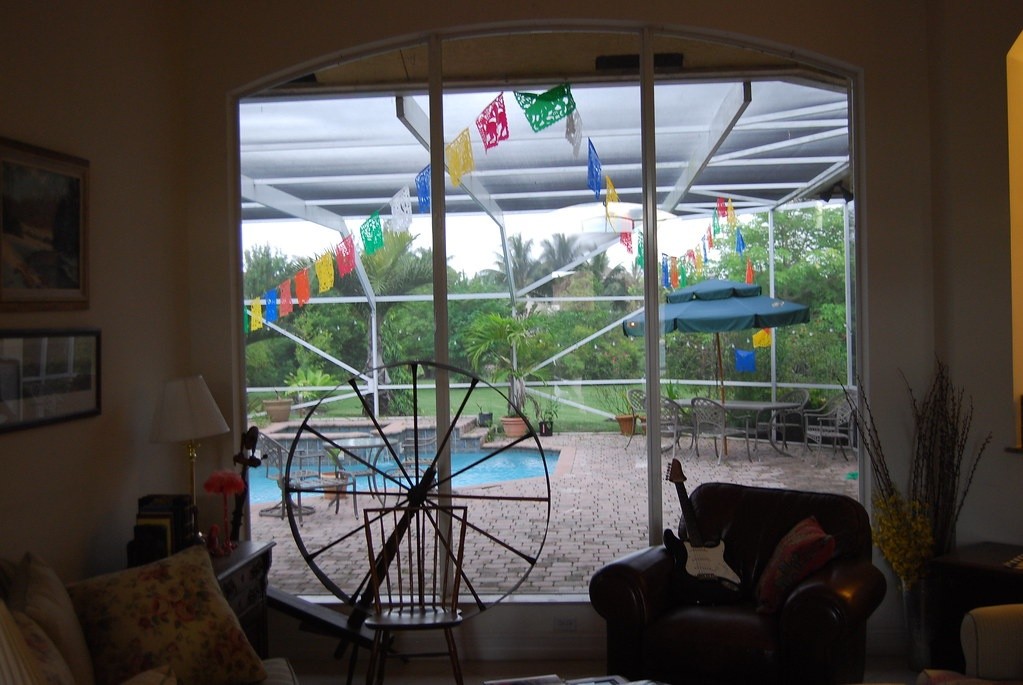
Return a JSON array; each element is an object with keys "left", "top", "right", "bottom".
[
  {"left": 670, "top": 398, "right": 813, "bottom": 462},
  {"left": 324, "top": 438, "right": 398, "bottom": 515},
  {"left": 927, "top": 541, "right": 1023, "bottom": 676}
]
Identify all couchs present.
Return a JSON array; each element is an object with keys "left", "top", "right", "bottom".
[
  {"left": 590, "top": 483, "right": 889, "bottom": 685},
  {"left": 0, "top": 599, "right": 302, "bottom": 685}
]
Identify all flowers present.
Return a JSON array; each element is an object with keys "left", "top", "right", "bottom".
[{"left": 871, "top": 486, "right": 935, "bottom": 589}]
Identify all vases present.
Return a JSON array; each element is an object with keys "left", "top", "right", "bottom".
[
  {"left": 639, "top": 416, "right": 647, "bottom": 435},
  {"left": 616, "top": 415, "right": 638, "bottom": 436},
  {"left": 901, "top": 576, "right": 933, "bottom": 671},
  {"left": 262, "top": 399, "right": 293, "bottom": 422}
]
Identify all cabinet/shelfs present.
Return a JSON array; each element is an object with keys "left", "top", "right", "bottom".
[{"left": 210, "top": 539, "right": 276, "bottom": 660}]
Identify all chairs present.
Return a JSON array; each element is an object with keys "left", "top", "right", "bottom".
[
  {"left": 258, "top": 433, "right": 437, "bottom": 527},
  {"left": 364, "top": 506, "right": 468, "bottom": 685},
  {"left": 625, "top": 388, "right": 858, "bottom": 468}
]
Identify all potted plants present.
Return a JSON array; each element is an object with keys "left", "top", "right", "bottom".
[
  {"left": 539, "top": 397, "right": 559, "bottom": 437},
  {"left": 499, "top": 412, "right": 531, "bottom": 437},
  {"left": 321, "top": 448, "right": 347, "bottom": 499}
]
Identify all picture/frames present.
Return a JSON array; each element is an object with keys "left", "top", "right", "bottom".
[
  {"left": 0, "top": 136, "right": 90, "bottom": 312},
  {"left": 0, "top": 326, "right": 102, "bottom": 434}
]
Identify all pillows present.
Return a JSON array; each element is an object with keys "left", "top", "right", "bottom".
[
  {"left": 756, "top": 516, "right": 839, "bottom": 610},
  {"left": 67, "top": 544, "right": 268, "bottom": 685},
  {"left": 0, "top": 558, "right": 176, "bottom": 685}
]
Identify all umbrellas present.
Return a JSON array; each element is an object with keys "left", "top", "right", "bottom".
[{"left": 622, "top": 278, "right": 810, "bottom": 457}]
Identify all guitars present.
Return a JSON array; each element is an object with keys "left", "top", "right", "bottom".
[{"left": 663, "top": 458, "right": 742, "bottom": 592}]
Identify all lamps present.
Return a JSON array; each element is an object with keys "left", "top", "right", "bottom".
[
  {"left": 821, "top": 180, "right": 854, "bottom": 204},
  {"left": 148, "top": 374, "right": 232, "bottom": 527}
]
